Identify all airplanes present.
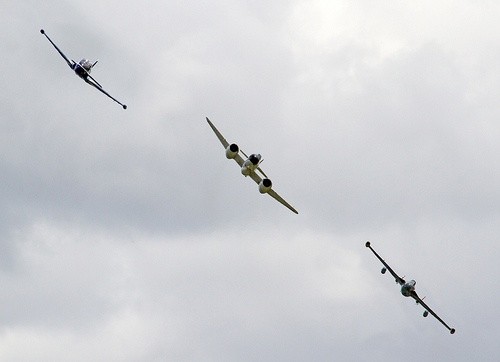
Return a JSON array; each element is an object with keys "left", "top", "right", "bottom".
[
  {"left": 39, "top": 29, "right": 127, "bottom": 109},
  {"left": 205, "top": 117, "right": 298, "bottom": 214},
  {"left": 366, "top": 241, "right": 455, "bottom": 334}
]
